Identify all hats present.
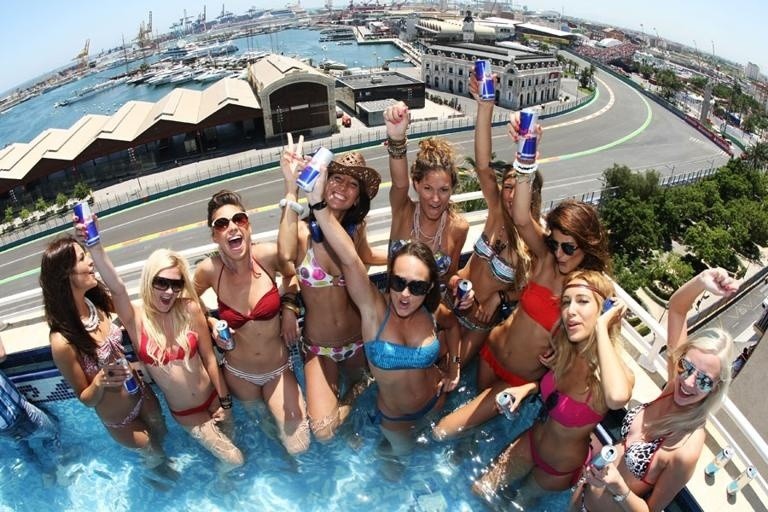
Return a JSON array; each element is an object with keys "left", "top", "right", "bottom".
[{"left": 327, "top": 151, "right": 382, "bottom": 201}]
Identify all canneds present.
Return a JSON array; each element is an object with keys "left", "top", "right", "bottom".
[
  {"left": 295, "top": 147, "right": 334, "bottom": 193},
  {"left": 591, "top": 444, "right": 618, "bottom": 470},
  {"left": 116, "top": 358, "right": 140, "bottom": 395},
  {"left": 73, "top": 201, "right": 100, "bottom": 247},
  {"left": 518, "top": 110, "right": 539, "bottom": 161},
  {"left": 704, "top": 447, "right": 733, "bottom": 478},
  {"left": 475, "top": 59, "right": 496, "bottom": 101},
  {"left": 458, "top": 279, "right": 473, "bottom": 299},
  {"left": 216, "top": 320, "right": 235, "bottom": 350},
  {"left": 727, "top": 466, "right": 757, "bottom": 496},
  {"left": 495, "top": 392, "right": 521, "bottom": 421}
]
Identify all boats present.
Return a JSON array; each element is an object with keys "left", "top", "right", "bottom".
[
  {"left": 384, "top": 55, "right": 404, "bottom": 63},
  {"left": 319, "top": 57, "right": 347, "bottom": 69},
  {"left": 316, "top": 26, "right": 354, "bottom": 42},
  {"left": 40, "top": 74, "right": 78, "bottom": 96},
  {"left": 335, "top": 39, "right": 354, "bottom": 45}
]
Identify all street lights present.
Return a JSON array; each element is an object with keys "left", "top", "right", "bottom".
[
  {"left": 7, "top": 188, "right": 23, "bottom": 224},
  {"left": 126, "top": 144, "right": 142, "bottom": 192},
  {"left": 352, "top": 60, "right": 358, "bottom": 68},
  {"left": 376, "top": 56, "right": 380, "bottom": 70},
  {"left": 276, "top": 104, "right": 285, "bottom": 153}
]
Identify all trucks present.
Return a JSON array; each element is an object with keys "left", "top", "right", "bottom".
[
  {"left": 335, "top": 106, "right": 343, "bottom": 118},
  {"left": 341, "top": 115, "right": 351, "bottom": 127}
]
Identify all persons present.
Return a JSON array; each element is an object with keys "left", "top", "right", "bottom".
[
  {"left": 37, "top": 66, "right": 740, "bottom": 512},
  {"left": 0, "top": 337, "right": 59, "bottom": 462}
]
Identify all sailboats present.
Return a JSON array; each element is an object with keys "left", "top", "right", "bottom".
[{"left": 51, "top": 1, "right": 273, "bottom": 113}]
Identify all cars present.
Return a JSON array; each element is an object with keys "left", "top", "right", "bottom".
[
  {"left": 717, "top": 133, "right": 722, "bottom": 138},
  {"left": 723, "top": 138, "right": 732, "bottom": 148}
]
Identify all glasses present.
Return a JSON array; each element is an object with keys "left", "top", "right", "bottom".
[
  {"left": 386, "top": 272, "right": 431, "bottom": 296},
  {"left": 151, "top": 275, "right": 186, "bottom": 294},
  {"left": 212, "top": 211, "right": 250, "bottom": 237},
  {"left": 676, "top": 357, "right": 718, "bottom": 394},
  {"left": 540, "top": 232, "right": 581, "bottom": 256},
  {"left": 536, "top": 388, "right": 560, "bottom": 425}
]
